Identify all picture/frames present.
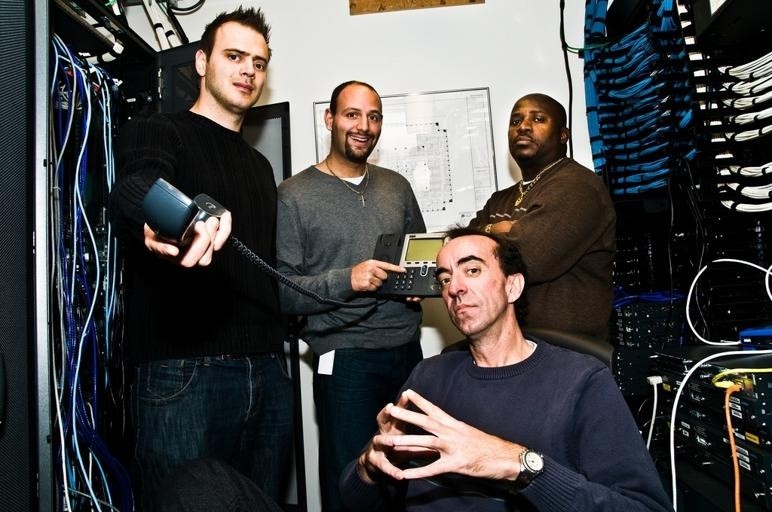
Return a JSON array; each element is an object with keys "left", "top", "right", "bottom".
[{"left": 313, "top": 86, "right": 498, "bottom": 233}]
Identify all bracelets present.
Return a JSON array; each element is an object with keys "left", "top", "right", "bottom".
[{"left": 486, "top": 224, "right": 494, "bottom": 232}]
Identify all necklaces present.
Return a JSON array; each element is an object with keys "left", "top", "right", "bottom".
[
  {"left": 514, "top": 163, "right": 554, "bottom": 206},
  {"left": 325, "top": 153, "right": 370, "bottom": 208}
]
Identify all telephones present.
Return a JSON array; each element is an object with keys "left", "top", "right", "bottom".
[{"left": 142, "top": 178, "right": 227, "bottom": 247}]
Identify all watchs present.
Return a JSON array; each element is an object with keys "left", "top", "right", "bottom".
[{"left": 510, "top": 444, "right": 545, "bottom": 491}]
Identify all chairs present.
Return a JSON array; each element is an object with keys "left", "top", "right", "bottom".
[{"left": 440, "top": 327, "right": 627, "bottom": 397}]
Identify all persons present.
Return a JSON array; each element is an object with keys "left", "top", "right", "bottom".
[
  {"left": 275, "top": 83, "right": 427, "bottom": 512},
  {"left": 340, "top": 228, "right": 678, "bottom": 512},
  {"left": 465, "top": 93, "right": 619, "bottom": 372},
  {"left": 111, "top": 9, "right": 296, "bottom": 511}
]
